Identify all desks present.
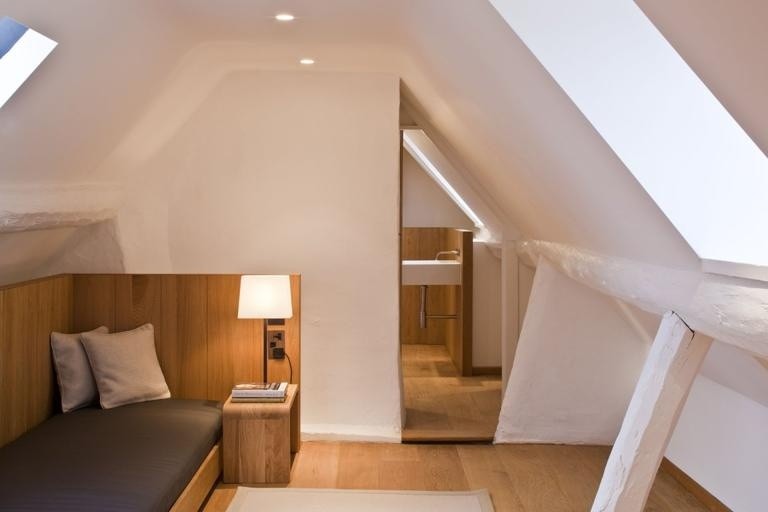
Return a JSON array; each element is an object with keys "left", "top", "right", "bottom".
[{"left": 222, "top": 383, "right": 301, "bottom": 487}]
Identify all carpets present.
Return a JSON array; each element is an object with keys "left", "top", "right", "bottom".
[{"left": 224, "top": 486, "right": 495, "bottom": 512}]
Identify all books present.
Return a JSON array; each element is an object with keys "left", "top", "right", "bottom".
[
  {"left": 232, "top": 382, "right": 288, "bottom": 397},
  {"left": 231, "top": 395, "right": 287, "bottom": 403}
]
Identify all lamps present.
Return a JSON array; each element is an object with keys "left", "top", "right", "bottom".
[{"left": 236, "top": 275, "right": 294, "bottom": 384}]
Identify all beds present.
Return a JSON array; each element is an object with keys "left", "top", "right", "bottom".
[{"left": 0, "top": 399, "right": 222, "bottom": 512}]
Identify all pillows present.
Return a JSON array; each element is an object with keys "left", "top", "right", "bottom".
[{"left": 50, "top": 322, "right": 172, "bottom": 414}]
